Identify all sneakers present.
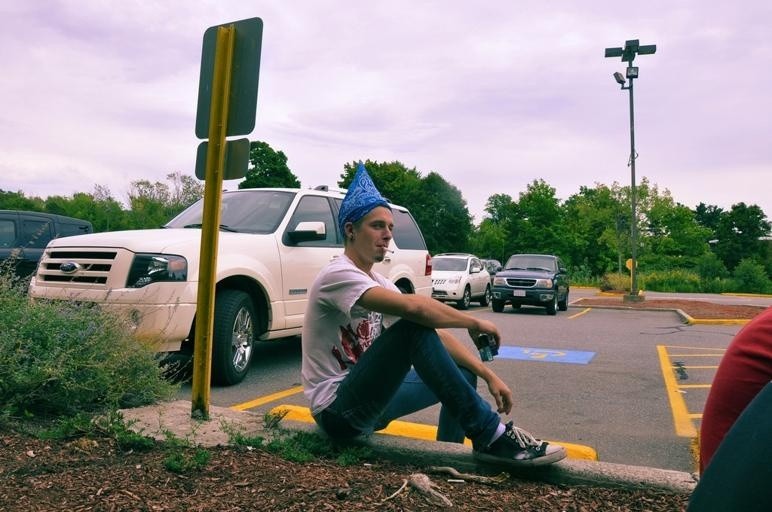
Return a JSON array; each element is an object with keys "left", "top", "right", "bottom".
[{"left": 472, "top": 420, "right": 567, "bottom": 468}]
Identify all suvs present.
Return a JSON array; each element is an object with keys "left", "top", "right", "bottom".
[
  {"left": 27, "top": 185, "right": 433, "bottom": 388},
  {"left": 491, "top": 254, "right": 568, "bottom": 315},
  {"left": 480, "top": 259, "right": 502, "bottom": 273},
  {"left": 0, "top": 210, "right": 94, "bottom": 281},
  {"left": 432, "top": 253, "right": 491, "bottom": 310}
]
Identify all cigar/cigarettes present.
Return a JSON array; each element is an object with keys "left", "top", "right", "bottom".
[{"left": 381, "top": 245, "right": 395, "bottom": 255}]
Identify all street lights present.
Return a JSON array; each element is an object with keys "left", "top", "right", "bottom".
[{"left": 604, "top": 39, "right": 657, "bottom": 302}]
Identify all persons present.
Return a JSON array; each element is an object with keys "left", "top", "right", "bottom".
[
  {"left": 300, "top": 162, "right": 568, "bottom": 468},
  {"left": 698, "top": 302, "right": 772, "bottom": 481}
]
